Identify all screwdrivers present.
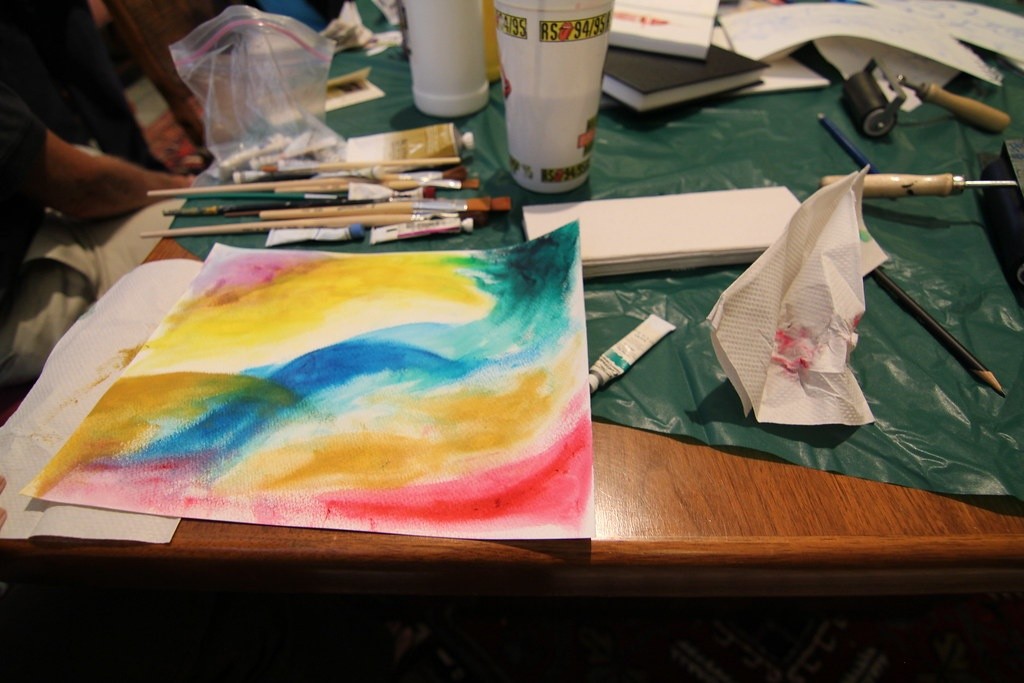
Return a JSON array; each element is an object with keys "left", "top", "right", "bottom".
[
  {"left": 821, "top": 174, "right": 1017, "bottom": 198},
  {"left": 897, "top": 75, "right": 1011, "bottom": 134}
]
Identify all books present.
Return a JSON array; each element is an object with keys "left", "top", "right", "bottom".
[
  {"left": 603, "top": 0, "right": 830, "bottom": 114},
  {"left": 522, "top": 186, "right": 800, "bottom": 281}
]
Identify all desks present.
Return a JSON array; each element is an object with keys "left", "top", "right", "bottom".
[{"left": 0, "top": 1, "right": 1024, "bottom": 683}]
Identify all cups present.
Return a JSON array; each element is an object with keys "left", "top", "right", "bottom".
[
  {"left": 492, "top": 0, "right": 617, "bottom": 193},
  {"left": 399, "top": 0, "right": 490, "bottom": 116}
]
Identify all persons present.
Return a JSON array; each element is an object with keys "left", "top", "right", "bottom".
[{"left": 0, "top": 0, "right": 374, "bottom": 384}]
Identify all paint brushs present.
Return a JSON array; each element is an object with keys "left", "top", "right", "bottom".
[{"left": 139, "top": 156, "right": 512, "bottom": 238}]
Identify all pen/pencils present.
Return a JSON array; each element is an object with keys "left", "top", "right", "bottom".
[
  {"left": 870, "top": 267, "right": 1007, "bottom": 398},
  {"left": 817, "top": 112, "right": 880, "bottom": 174}
]
